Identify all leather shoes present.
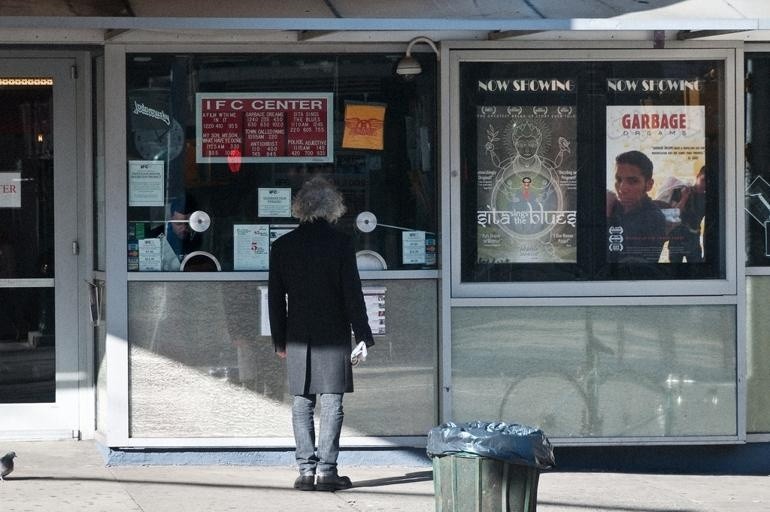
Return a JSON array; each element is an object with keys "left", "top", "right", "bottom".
[
  {"left": 294, "top": 476, "right": 314, "bottom": 490},
  {"left": 317, "top": 474, "right": 352, "bottom": 492}
]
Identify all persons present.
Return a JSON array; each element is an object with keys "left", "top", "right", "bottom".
[
  {"left": 267, "top": 175, "right": 374, "bottom": 492},
  {"left": 154, "top": 197, "right": 206, "bottom": 263},
  {"left": 668, "top": 164, "right": 706, "bottom": 263},
  {"left": 606, "top": 151, "right": 667, "bottom": 263},
  {"left": 520, "top": 174, "right": 533, "bottom": 194},
  {"left": 482, "top": 120, "right": 571, "bottom": 205}
]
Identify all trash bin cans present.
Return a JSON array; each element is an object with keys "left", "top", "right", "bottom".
[{"left": 427, "top": 421, "right": 544, "bottom": 512}]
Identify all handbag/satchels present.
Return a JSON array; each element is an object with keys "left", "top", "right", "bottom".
[{"left": 350, "top": 341, "right": 367, "bottom": 364}]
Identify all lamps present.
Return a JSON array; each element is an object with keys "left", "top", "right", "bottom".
[{"left": 392, "top": 34, "right": 444, "bottom": 79}]
